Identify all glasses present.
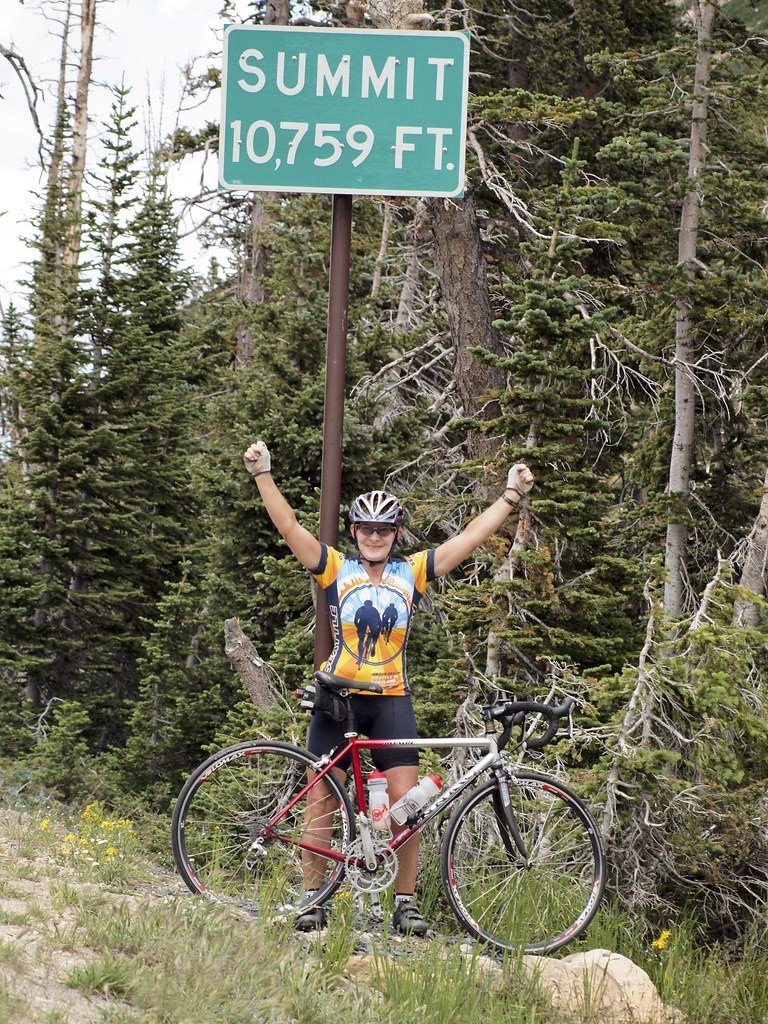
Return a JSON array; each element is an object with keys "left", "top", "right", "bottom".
[{"left": 355, "top": 524, "right": 396, "bottom": 536}]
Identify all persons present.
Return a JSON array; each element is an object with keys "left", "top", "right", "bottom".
[{"left": 244, "top": 442, "right": 535, "bottom": 934}]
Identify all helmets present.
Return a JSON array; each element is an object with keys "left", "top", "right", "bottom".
[{"left": 349, "top": 490, "right": 405, "bottom": 523}]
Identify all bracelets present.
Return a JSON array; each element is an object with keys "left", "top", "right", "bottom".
[{"left": 501, "top": 494, "right": 517, "bottom": 508}]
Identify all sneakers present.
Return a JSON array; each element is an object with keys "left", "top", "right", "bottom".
[
  {"left": 393, "top": 901, "right": 427, "bottom": 934},
  {"left": 294, "top": 906, "right": 326, "bottom": 931}
]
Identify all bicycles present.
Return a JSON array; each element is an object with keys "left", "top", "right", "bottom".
[{"left": 172, "top": 697, "right": 606, "bottom": 956}]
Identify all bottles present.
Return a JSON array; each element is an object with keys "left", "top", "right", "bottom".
[
  {"left": 367, "top": 770, "right": 391, "bottom": 830},
  {"left": 390, "top": 772, "right": 442, "bottom": 827}
]
